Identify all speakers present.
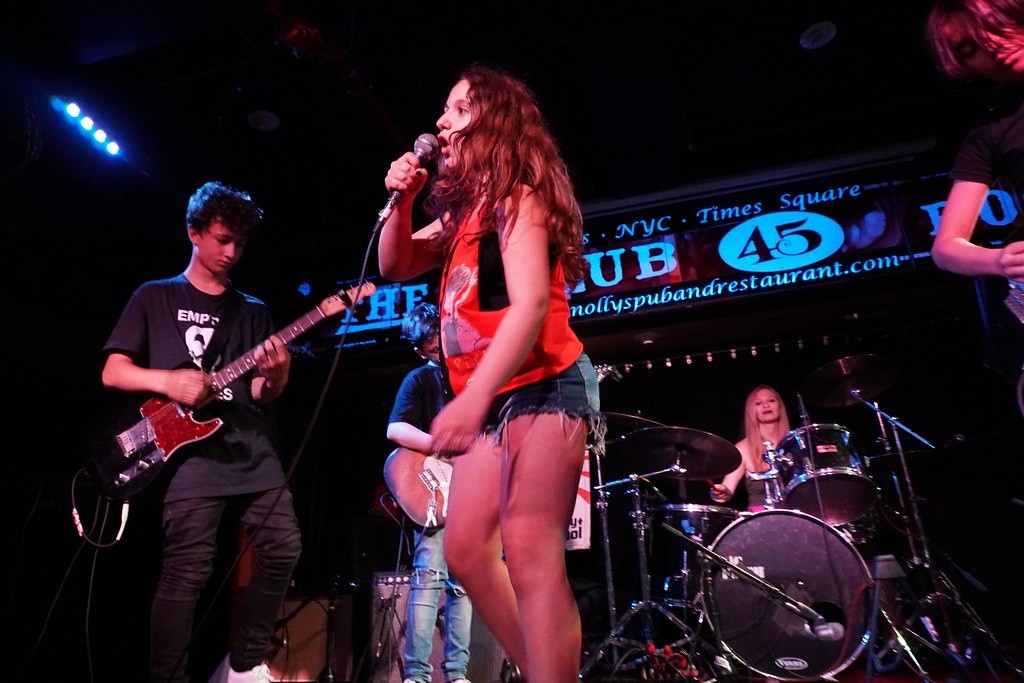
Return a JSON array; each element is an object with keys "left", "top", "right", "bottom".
[
  {"left": 207, "top": 594, "right": 352, "bottom": 683},
  {"left": 371, "top": 572, "right": 509, "bottom": 683}
]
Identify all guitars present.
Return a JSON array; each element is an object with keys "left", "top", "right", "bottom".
[
  {"left": 382, "top": 362, "right": 635, "bottom": 537},
  {"left": 78, "top": 279, "right": 375, "bottom": 501}
]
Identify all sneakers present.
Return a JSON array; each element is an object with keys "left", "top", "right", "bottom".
[{"left": 208, "top": 652, "right": 274, "bottom": 683}]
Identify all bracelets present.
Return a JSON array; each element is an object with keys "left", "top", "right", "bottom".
[{"left": 262, "top": 379, "right": 270, "bottom": 401}]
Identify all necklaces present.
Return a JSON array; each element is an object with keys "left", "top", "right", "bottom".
[{"left": 763, "top": 437, "right": 777, "bottom": 445}]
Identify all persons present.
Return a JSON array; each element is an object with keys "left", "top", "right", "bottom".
[
  {"left": 927, "top": 0, "right": 1023, "bottom": 287},
  {"left": 709, "top": 384, "right": 792, "bottom": 513},
  {"left": 378, "top": 60, "right": 599, "bottom": 683},
  {"left": 101, "top": 180, "right": 301, "bottom": 683}
]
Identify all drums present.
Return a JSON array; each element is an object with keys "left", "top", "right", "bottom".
[
  {"left": 646, "top": 500, "right": 740, "bottom": 610},
  {"left": 770, "top": 420, "right": 879, "bottom": 528},
  {"left": 696, "top": 507, "right": 879, "bottom": 683}
]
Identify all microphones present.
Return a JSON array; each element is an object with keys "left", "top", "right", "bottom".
[
  {"left": 374, "top": 134, "right": 439, "bottom": 234},
  {"left": 811, "top": 622, "right": 845, "bottom": 641},
  {"left": 270, "top": 634, "right": 291, "bottom": 649}
]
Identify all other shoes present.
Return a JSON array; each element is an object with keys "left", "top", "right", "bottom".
[
  {"left": 403, "top": 678, "right": 416, "bottom": 683},
  {"left": 453, "top": 678, "right": 471, "bottom": 683}
]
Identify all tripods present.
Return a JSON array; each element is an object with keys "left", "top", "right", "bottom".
[
  {"left": 845, "top": 382, "right": 1024, "bottom": 683},
  {"left": 579, "top": 428, "right": 723, "bottom": 683}
]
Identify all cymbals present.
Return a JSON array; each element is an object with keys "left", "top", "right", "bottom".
[
  {"left": 600, "top": 411, "right": 667, "bottom": 429},
  {"left": 617, "top": 424, "right": 743, "bottom": 481},
  {"left": 799, "top": 351, "right": 899, "bottom": 407},
  {"left": 869, "top": 447, "right": 936, "bottom": 462}
]
